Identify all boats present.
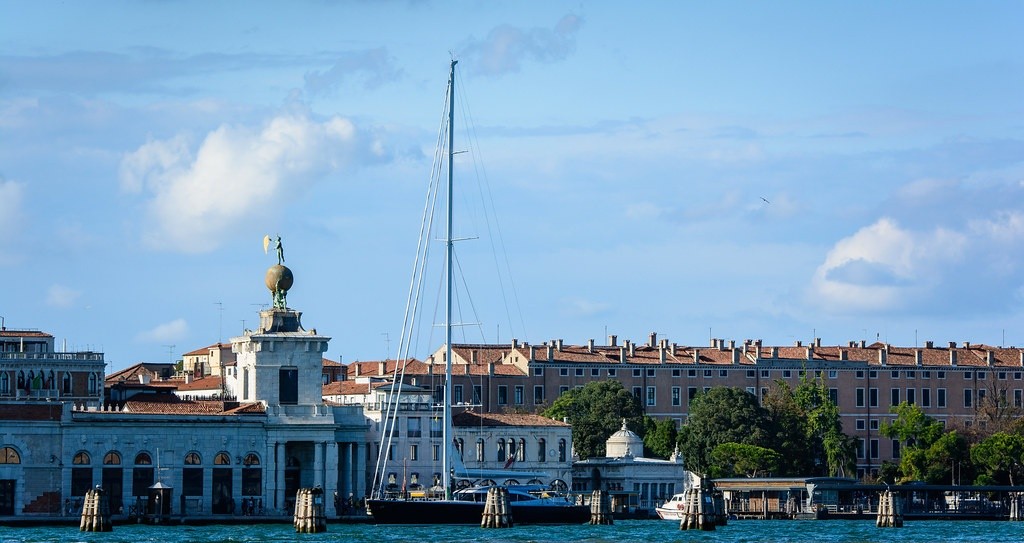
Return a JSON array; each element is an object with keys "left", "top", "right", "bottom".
[{"left": 655, "top": 494, "right": 728, "bottom": 524}]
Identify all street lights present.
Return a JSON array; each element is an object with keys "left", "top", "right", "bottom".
[
  {"left": 959, "top": 461, "right": 962, "bottom": 485},
  {"left": 950, "top": 458, "right": 955, "bottom": 486}
]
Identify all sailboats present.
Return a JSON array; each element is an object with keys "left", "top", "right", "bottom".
[{"left": 364, "top": 59, "right": 592, "bottom": 526}]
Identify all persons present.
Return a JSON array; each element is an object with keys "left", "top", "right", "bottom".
[
  {"left": 270, "top": 236, "right": 284, "bottom": 265},
  {"left": 275, "top": 281, "right": 287, "bottom": 308}
]
